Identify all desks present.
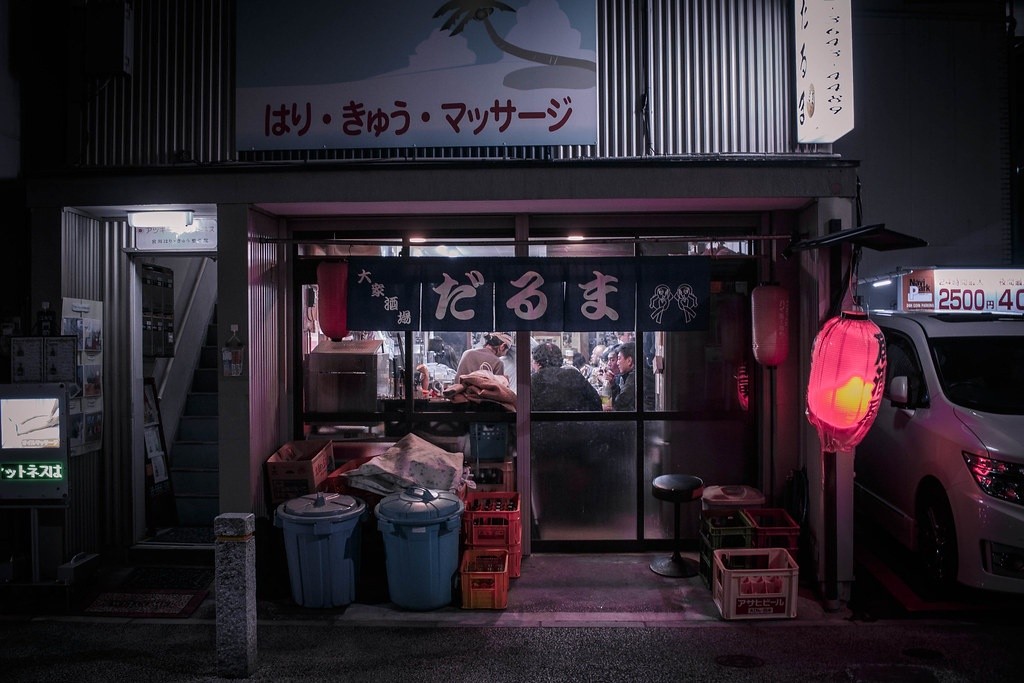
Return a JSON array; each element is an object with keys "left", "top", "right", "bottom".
[{"left": 383, "top": 394, "right": 505, "bottom": 438}]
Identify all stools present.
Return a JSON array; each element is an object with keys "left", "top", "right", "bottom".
[{"left": 650, "top": 473, "right": 703, "bottom": 578}]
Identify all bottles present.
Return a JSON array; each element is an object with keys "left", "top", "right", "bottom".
[
  {"left": 466, "top": 468, "right": 515, "bottom": 525},
  {"left": 465, "top": 558, "right": 505, "bottom": 588}
]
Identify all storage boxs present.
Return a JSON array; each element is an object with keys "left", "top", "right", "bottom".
[
  {"left": 462, "top": 422, "right": 523, "bottom": 610},
  {"left": 698, "top": 508, "right": 801, "bottom": 589},
  {"left": 266, "top": 440, "right": 336, "bottom": 496},
  {"left": 326, "top": 454, "right": 383, "bottom": 512},
  {"left": 712, "top": 548, "right": 799, "bottom": 620}
]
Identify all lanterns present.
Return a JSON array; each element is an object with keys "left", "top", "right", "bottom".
[
  {"left": 317, "top": 259, "right": 349, "bottom": 342},
  {"left": 739, "top": 362, "right": 749, "bottom": 411},
  {"left": 805, "top": 311, "right": 886, "bottom": 452},
  {"left": 751, "top": 282, "right": 790, "bottom": 370}
]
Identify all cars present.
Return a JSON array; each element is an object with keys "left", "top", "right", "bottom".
[{"left": 856, "top": 307, "right": 1024, "bottom": 600}]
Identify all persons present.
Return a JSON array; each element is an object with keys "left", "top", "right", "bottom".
[{"left": 435, "top": 332, "right": 655, "bottom": 524}]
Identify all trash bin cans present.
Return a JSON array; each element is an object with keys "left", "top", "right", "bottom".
[
  {"left": 374, "top": 483, "right": 466, "bottom": 611},
  {"left": 274, "top": 490, "right": 369, "bottom": 616}
]
